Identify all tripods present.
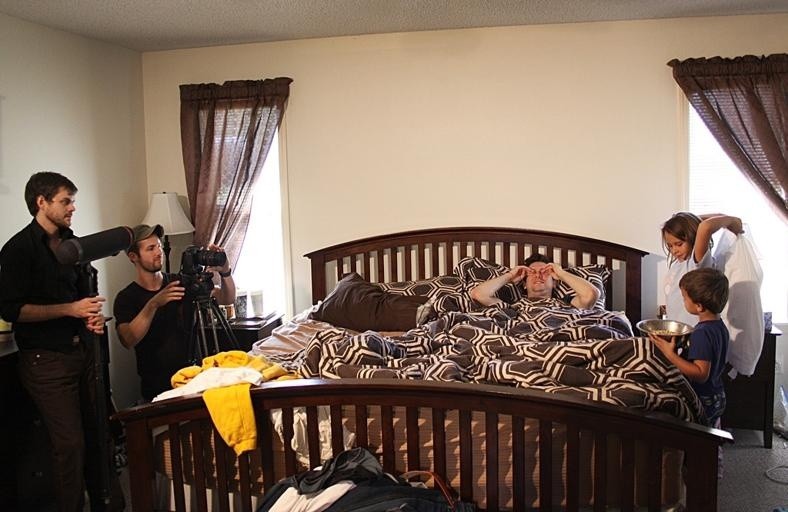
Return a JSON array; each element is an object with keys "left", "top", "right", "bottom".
[{"left": 190, "top": 298, "right": 240, "bottom": 358}]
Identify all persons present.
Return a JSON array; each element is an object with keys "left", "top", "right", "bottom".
[
  {"left": 113, "top": 219, "right": 237, "bottom": 405},
  {"left": 657, "top": 209, "right": 745, "bottom": 323},
  {"left": 649, "top": 269, "right": 728, "bottom": 481},
  {"left": 0, "top": 169, "right": 123, "bottom": 511},
  {"left": 469, "top": 252, "right": 602, "bottom": 311}
]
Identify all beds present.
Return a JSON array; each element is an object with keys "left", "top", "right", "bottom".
[{"left": 109, "top": 225, "right": 735, "bottom": 511}]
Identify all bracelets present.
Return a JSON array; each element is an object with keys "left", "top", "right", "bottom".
[{"left": 218, "top": 267, "right": 231, "bottom": 278}]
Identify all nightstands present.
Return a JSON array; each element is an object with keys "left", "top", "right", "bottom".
[
  {"left": 721, "top": 323, "right": 784, "bottom": 450},
  {"left": 200, "top": 311, "right": 286, "bottom": 358}
]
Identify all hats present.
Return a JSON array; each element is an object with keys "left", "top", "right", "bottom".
[{"left": 124, "top": 224, "right": 164, "bottom": 256}]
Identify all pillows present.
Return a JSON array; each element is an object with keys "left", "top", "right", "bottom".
[{"left": 714, "top": 228, "right": 766, "bottom": 377}]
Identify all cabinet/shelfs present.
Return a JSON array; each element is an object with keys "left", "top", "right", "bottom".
[{"left": 1, "top": 316, "right": 113, "bottom": 512}]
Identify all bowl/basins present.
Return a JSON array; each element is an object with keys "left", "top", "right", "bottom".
[{"left": 636, "top": 318, "right": 694, "bottom": 346}]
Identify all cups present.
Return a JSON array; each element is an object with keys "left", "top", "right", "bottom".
[
  {"left": 218, "top": 305, "right": 234, "bottom": 321},
  {"left": 234, "top": 292, "right": 247, "bottom": 317},
  {"left": 251, "top": 291, "right": 265, "bottom": 315},
  {"left": 764, "top": 312, "right": 772, "bottom": 332}
]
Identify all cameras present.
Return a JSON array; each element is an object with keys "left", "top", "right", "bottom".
[{"left": 175, "top": 244, "right": 226, "bottom": 299}]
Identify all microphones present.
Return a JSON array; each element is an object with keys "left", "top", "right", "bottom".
[{"left": 55, "top": 225, "right": 136, "bottom": 266}]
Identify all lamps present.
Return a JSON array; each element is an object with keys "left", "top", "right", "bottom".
[{"left": 138, "top": 191, "right": 198, "bottom": 274}]
